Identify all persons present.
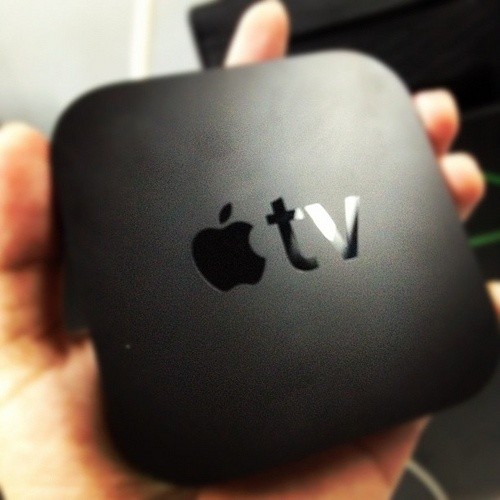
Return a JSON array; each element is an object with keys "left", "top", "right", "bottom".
[{"left": 0, "top": 0, "right": 500, "bottom": 500}]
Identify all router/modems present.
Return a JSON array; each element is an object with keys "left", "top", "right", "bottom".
[{"left": 50, "top": 49, "right": 499, "bottom": 483}]
